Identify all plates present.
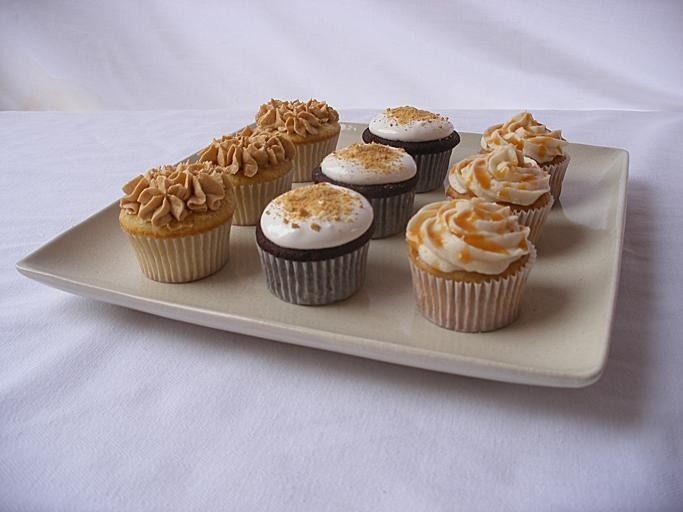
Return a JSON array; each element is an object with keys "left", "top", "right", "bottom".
[{"left": 14, "top": 118, "right": 630, "bottom": 389}]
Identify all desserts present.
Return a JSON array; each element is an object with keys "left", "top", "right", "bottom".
[{"left": 116, "top": 98, "right": 568, "bottom": 333}]
